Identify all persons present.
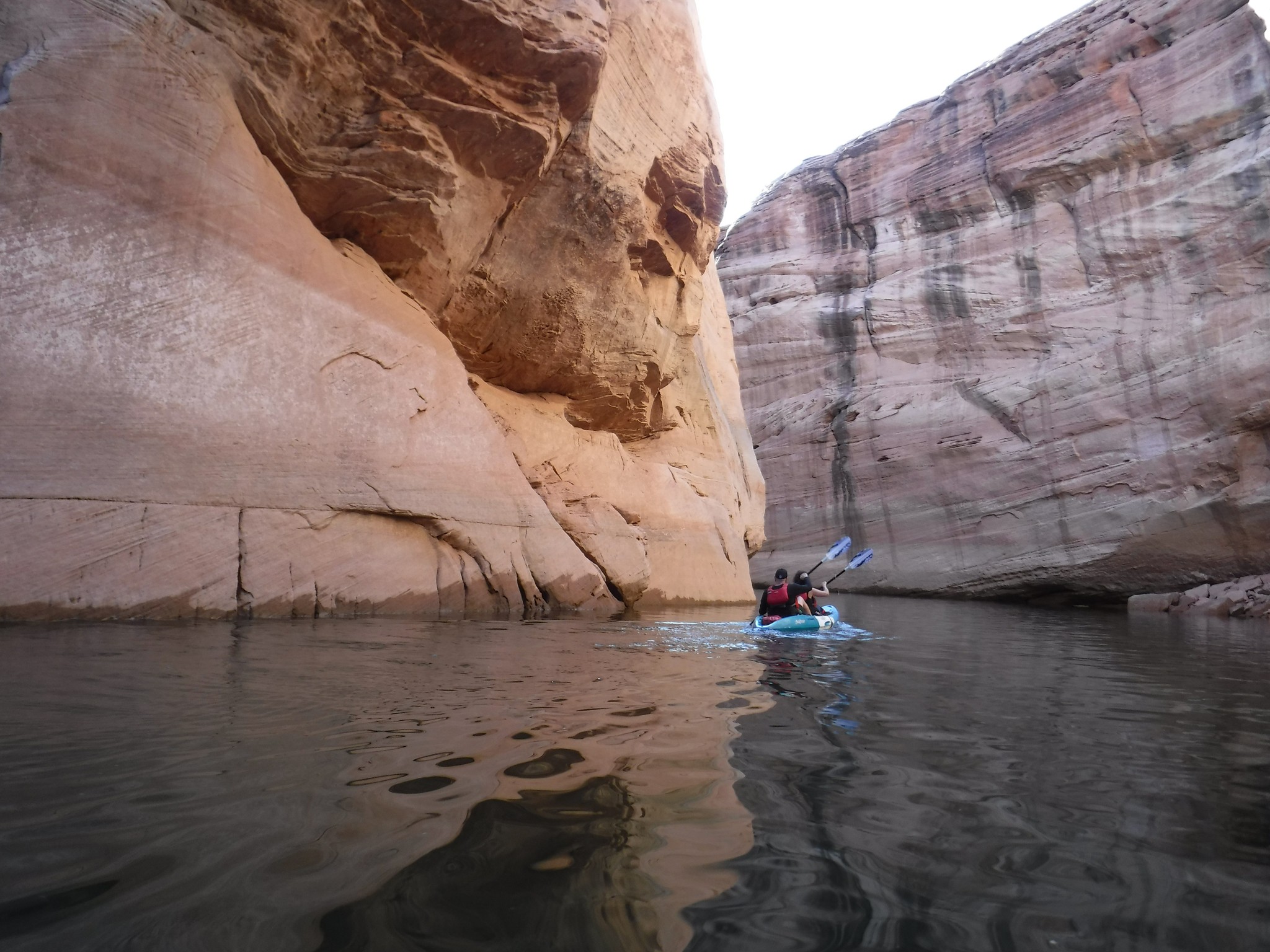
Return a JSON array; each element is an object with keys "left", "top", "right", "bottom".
[{"left": 759, "top": 568, "right": 829, "bottom": 618}]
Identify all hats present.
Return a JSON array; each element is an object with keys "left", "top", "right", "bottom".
[{"left": 776, "top": 568, "right": 788, "bottom": 579}]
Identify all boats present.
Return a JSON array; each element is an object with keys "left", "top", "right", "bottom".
[{"left": 754, "top": 604, "right": 840, "bottom": 631}]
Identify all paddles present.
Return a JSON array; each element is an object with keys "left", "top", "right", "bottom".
[
  {"left": 748, "top": 534, "right": 852, "bottom": 617},
  {"left": 812, "top": 547, "right": 874, "bottom": 595}
]
